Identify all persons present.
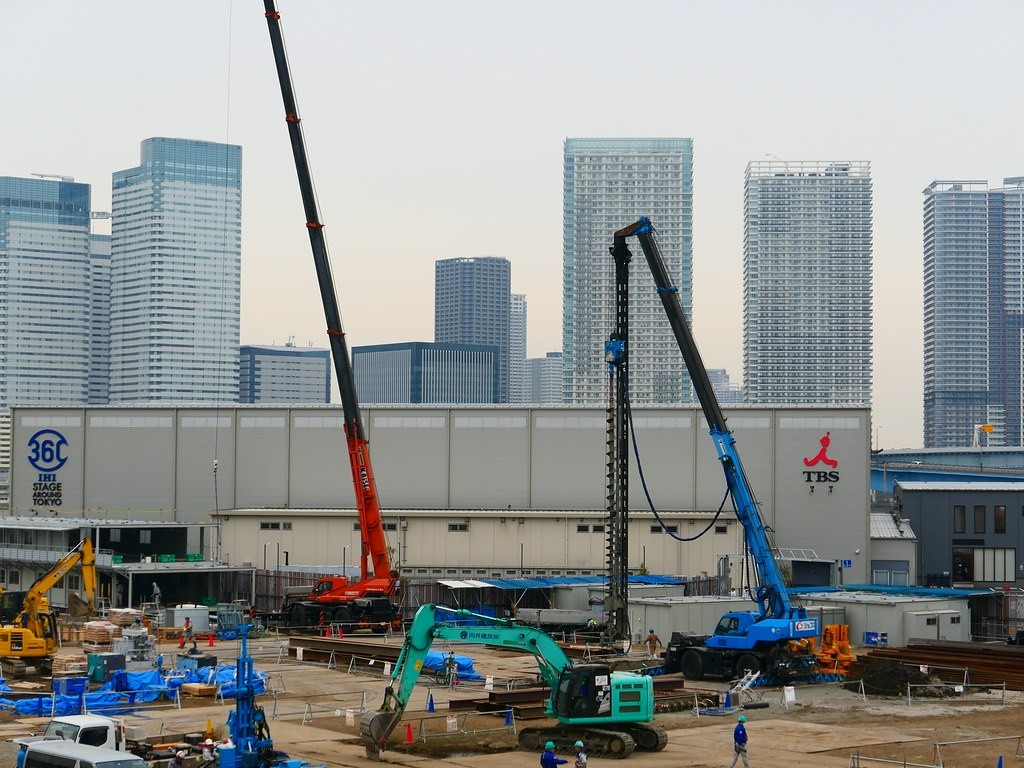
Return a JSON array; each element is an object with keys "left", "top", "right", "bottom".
[
  {"left": 730, "top": 716, "right": 750, "bottom": 768},
  {"left": 731, "top": 588, "right": 736, "bottom": 596},
  {"left": 643, "top": 628, "right": 662, "bottom": 659},
  {"left": 116, "top": 580, "right": 123, "bottom": 606},
  {"left": 540, "top": 742, "right": 571, "bottom": 768},
  {"left": 586, "top": 618, "right": 598, "bottom": 628},
  {"left": 743, "top": 586, "right": 750, "bottom": 598},
  {"left": 250, "top": 606, "right": 255, "bottom": 618},
  {"left": 150, "top": 582, "right": 161, "bottom": 604},
  {"left": 575, "top": 741, "right": 586, "bottom": 768},
  {"left": 168, "top": 751, "right": 185, "bottom": 768},
  {"left": 203, "top": 739, "right": 215, "bottom": 760},
  {"left": 181, "top": 617, "right": 197, "bottom": 648},
  {"left": 130, "top": 616, "right": 145, "bottom": 628},
  {"left": 16, "top": 745, "right": 26, "bottom": 768}
]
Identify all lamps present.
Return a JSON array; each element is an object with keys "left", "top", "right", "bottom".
[
  {"left": 726, "top": 520, "right": 730, "bottom": 523},
  {"left": 809, "top": 485, "right": 814, "bottom": 496},
  {"left": 629, "top": 519, "right": 632, "bottom": 521},
  {"left": 828, "top": 485, "right": 833, "bottom": 494},
  {"left": 464, "top": 519, "right": 469, "bottom": 521},
  {"left": 690, "top": 520, "right": 693, "bottom": 523},
  {"left": 225, "top": 516, "right": 229, "bottom": 520},
  {"left": 500, "top": 518, "right": 504, "bottom": 523},
  {"left": 400, "top": 517, "right": 405, "bottom": 521},
  {"left": 519, "top": 518, "right": 524, "bottom": 523}
]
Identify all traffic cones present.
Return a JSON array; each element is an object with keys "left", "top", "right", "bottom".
[
  {"left": 206, "top": 720, "right": 213, "bottom": 733},
  {"left": 725, "top": 691, "right": 731, "bottom": 707},
  {"left": 325, "top": 627, "right": 330, "bottom": 637},
  {"left": 403, "top": 723, "right": 416, "bottom": 744},
  {"left": 178, "top": 636, "right": 187, "bottom": 648},
  {"left": 207, "top": 635, "right": 216, "bottom": 647},
  {"left": 339, "top": 628, "right": 343, "bottom": 639},
  {"left": 997, "top": 755, "right": 1003, "bottom": 768},
  {"left": 504, "top": 706, "right": 514, "bottom": 725},
  {"left": 428, "top": 694, "right": 436, "bottom": 713}
]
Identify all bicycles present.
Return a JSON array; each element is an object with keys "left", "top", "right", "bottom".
[{"left": 436, "top": 651, "right": 459, "bottom": 687}]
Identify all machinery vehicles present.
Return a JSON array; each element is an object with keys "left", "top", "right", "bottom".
[
  {"left": 264, "top": 0, "right": 404, "bottom": 637},
  {"left": 0, "top": 537, "right": 98, "bottom": 680},
  {"left": 360, "top": 603, "right": 668, "bottom": 762},
  {"left": 603, "top": 214, "right": 822, "bottom": 691}
]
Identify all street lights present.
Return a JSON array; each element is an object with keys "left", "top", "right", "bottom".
[{"left": 877, "top": 426, "right": 882, "bottom": 450}]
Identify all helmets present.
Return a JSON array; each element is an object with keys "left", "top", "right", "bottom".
[
  {"left": 136, "top": 616, "right": 141, "bottom": 620},
  {"left": 738, "top": 716, "right": 746, "bottom": 722},
  {"left": 649, "top": 628, "right": 654, "bottom": 632},
  {"left": 574, "top": 741, "right": 583, "bottom": 747},
  {"left": 185, "top": 617, "right": 189, "bottom": 621},
  {"left": 545, "top": 741, "right": 555, "bottom": 748},
  {"left": 587, "top": 617, "right": 591, "bottom": 622}
]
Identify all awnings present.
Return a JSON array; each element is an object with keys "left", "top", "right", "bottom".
[{"left": 436, "top": 574, "right": 685, "bottom": 590}]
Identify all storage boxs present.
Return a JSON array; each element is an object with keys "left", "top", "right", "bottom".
[
  {"left": 200, "top": 596, "right": 218, "bottom": 605},
  {"left": 113, "top": 555, "right": 123, "bottom": 563},
  {"left": 159, "top": 554, "right": 175, "bottom": 563},
  {"left": 185, "top": 553, "right": 204, "bottom": 561}
]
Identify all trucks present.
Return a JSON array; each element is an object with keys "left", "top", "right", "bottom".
[{"left": 43, "top": 712, "right": 219, "bottom": 768}]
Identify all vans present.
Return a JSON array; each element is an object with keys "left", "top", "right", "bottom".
[{"left": 5, "top": 736, "right": 148, "bottom": 768}]
